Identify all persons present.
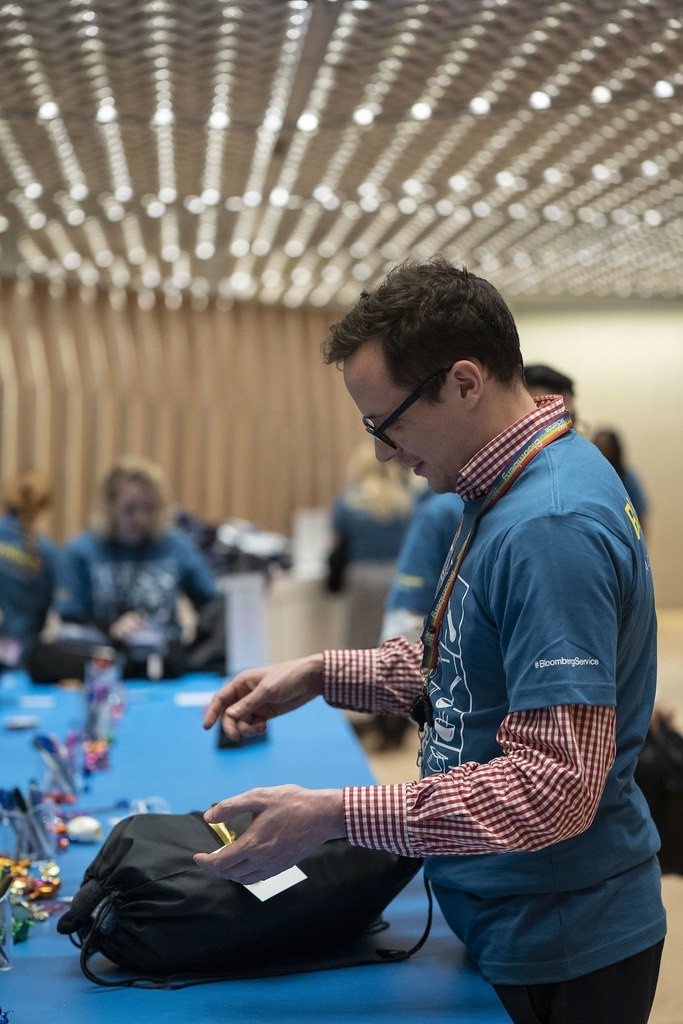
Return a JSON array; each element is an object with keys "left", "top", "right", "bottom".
[
  {"left": 382, "top": 364, "right": 574, "bottom": 646},
  {"left": 1, "top": 457, "right": 223, "bottom": 678},
  {"left": 592, "top": 429, "right": 647, "bottom": 539},
  {"left": 194, "top": 261, "right": 668, "bottom": 1024},
  {"left": 332, "top": 438, "right": 428, "bottom": 749}
]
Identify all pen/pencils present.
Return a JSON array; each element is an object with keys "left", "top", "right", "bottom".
[
  {"left": 30, "top": 728, "right": 87, "bottom": 796},
  {"left": 0, "top": 779, "right": 60, "bottom": 863},
  {"left": 0, "top": 863, "right": 16, "bottom": 973}
]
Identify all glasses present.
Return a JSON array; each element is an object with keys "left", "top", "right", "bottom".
[{"left": 362, "top": 365, "right": 453, "bottom": 450}]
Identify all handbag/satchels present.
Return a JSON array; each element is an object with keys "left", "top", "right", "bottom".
[{"left": 55, "top": 808, "right": 434, "bottom": 991}]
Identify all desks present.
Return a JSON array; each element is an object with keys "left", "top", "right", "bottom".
[{"left": 0, "top": 674, "right": 514, "bottom": 1024}]
